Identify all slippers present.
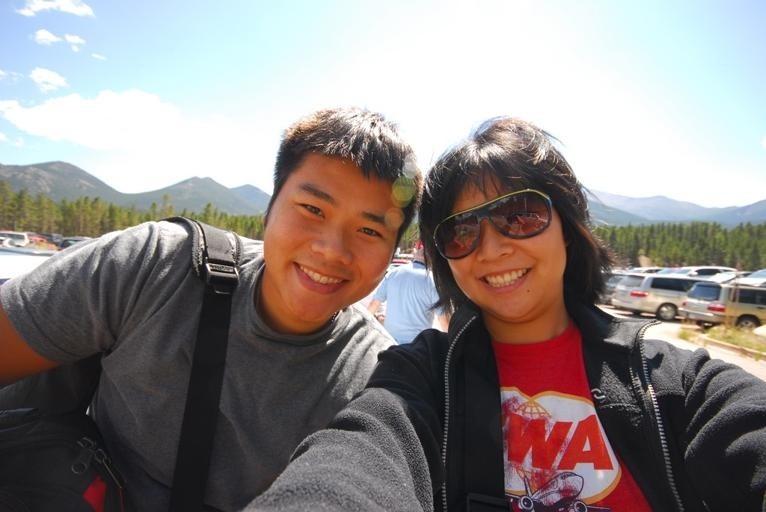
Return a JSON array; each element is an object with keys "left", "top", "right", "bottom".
[{"left": 0, "top": 356, "right": 131, "bottom": 510}]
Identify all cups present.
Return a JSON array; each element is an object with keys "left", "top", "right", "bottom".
[{"left": 433, "top": 188, "right": 552, "bottom": 260}]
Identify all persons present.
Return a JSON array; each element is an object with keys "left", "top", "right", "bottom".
[
  {"left": 240, "top": 115, "right": 766, "bottom": 512},
  {"left": 367, "top": 245, "right": 452, "bottom": 345},
  {"left": 0, "top": 107, "right": 424, "bottom": 512}
]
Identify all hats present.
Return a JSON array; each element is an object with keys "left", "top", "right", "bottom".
[
  {"left": 601, "top": 265, "right": 766, "bottom": 329},
  {"left": 1, "top": 229, "right": 93, "bottom": 249}
]
